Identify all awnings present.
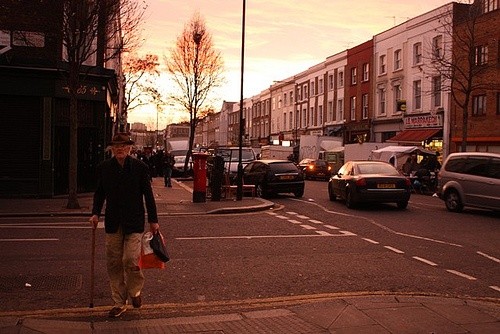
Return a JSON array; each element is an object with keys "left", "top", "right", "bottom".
[{"left": 386, "top": 127, "right": 442, "bottom": 145}]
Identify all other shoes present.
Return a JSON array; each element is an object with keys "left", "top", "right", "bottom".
[
  {"left": 168, "top": 185, "right": 172, "bottom": 187},
  {"left": 109, "top": 307, "right": 127, "bottom": 318},
  {"left": 132, "top": 296, "right": 143, "bottom": 308}
]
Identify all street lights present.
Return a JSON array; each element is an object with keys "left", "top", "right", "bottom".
[
  {"left": 157, "top": 104, "right": 175, "bottom": 153},
  {"left": 273, "top": 81, "right": 297, "bottom": 146}
]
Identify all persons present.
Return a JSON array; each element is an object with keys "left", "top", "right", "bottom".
[
  {"left": 137, "top": 147, "right": 164, "bottom": 182},
  {"left": 89, "top": 132, "right": 159, "bottom": 317},
  {"left": 162, "top": 154, "right": 172, "bottom": 187},
  {"left": 402, "top": 155, "right": 442, "bottom": 186}
]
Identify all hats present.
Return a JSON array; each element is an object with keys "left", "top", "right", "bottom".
[{"left": 108, "top": 132, "right": 134, "bottom": 145}]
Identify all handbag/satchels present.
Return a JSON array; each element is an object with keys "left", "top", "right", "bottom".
[{"left": 138, "top": 229, "right": 171, "bottom": 270}]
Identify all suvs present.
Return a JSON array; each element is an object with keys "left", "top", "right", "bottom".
[{"left": 435, "top": 153, "right": 500, "bottom": 213}]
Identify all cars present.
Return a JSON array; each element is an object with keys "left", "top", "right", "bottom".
[
  {"left": 297, "top": 159, "right": 331, "bottom": 180},
  {"left": 328, "top": 160, "right": 412, "bottom": 210},
  {"left": 231, "top": 160, "right": 304, "bottom": 199}
]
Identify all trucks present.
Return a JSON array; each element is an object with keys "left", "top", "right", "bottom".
[{"left": 163, "top": 138, "right": 293, "bottom": 184}]
